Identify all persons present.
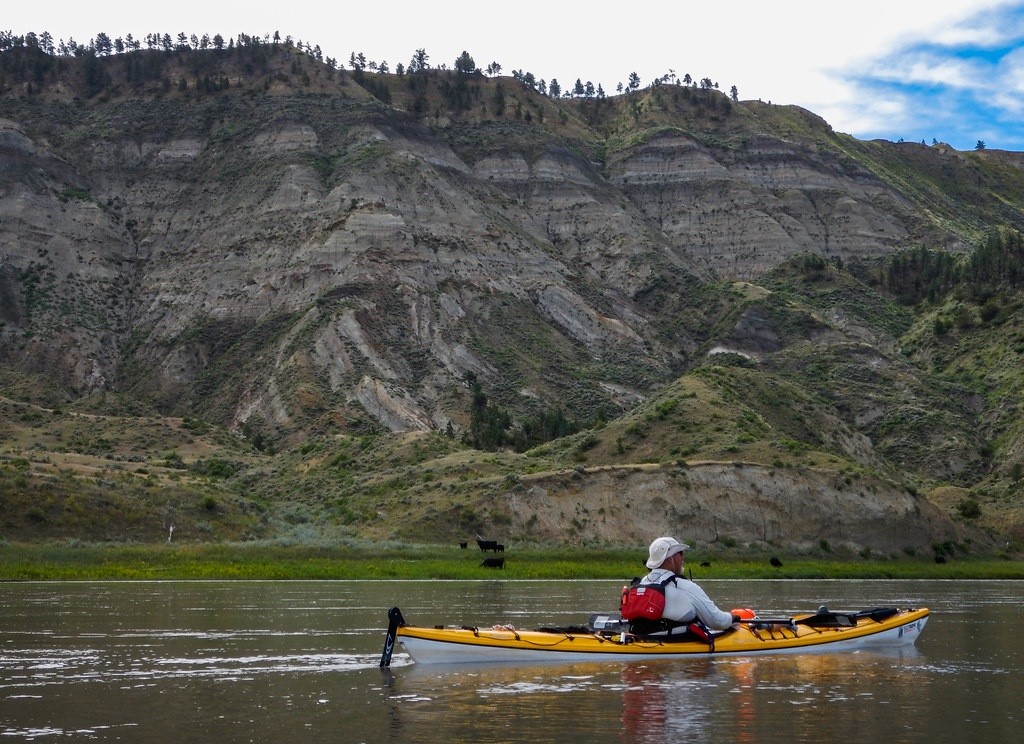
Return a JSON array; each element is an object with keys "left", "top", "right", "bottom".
[{"left": 629, "top": 537, "right": 741, "bottom": 643}]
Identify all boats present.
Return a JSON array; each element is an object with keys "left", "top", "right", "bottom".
[{"left": 379, "top": 603, "right": 930, "bottom": 671}]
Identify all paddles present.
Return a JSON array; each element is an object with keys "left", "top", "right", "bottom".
[{"left": 588, "top": 613, "right": 858, "bottom": 631}]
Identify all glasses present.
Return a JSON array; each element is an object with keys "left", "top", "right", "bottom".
[{"left": 677, "top": 551, "right": 683, "bottom": 557}]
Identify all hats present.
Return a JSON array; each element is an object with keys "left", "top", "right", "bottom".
[{"left": 646, "top": 537, "right": 690, "bottom": 569}]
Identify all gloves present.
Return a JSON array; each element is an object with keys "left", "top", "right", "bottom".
[{"left": 731, "top": 614, "right": 740, "bottom": 624}]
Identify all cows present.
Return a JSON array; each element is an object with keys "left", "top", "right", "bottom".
[
  {"left": 475, "top": 539, "right": 505, "bottom": 554},
  {"left": 458, "top": 541, "right": 467, "bottom": 550},
  {"left": 479, "top": 557, "right": 507, "bottom": 570},
  {"left": 699, "top": 562, "right": 712, "bottom": 569}
]
